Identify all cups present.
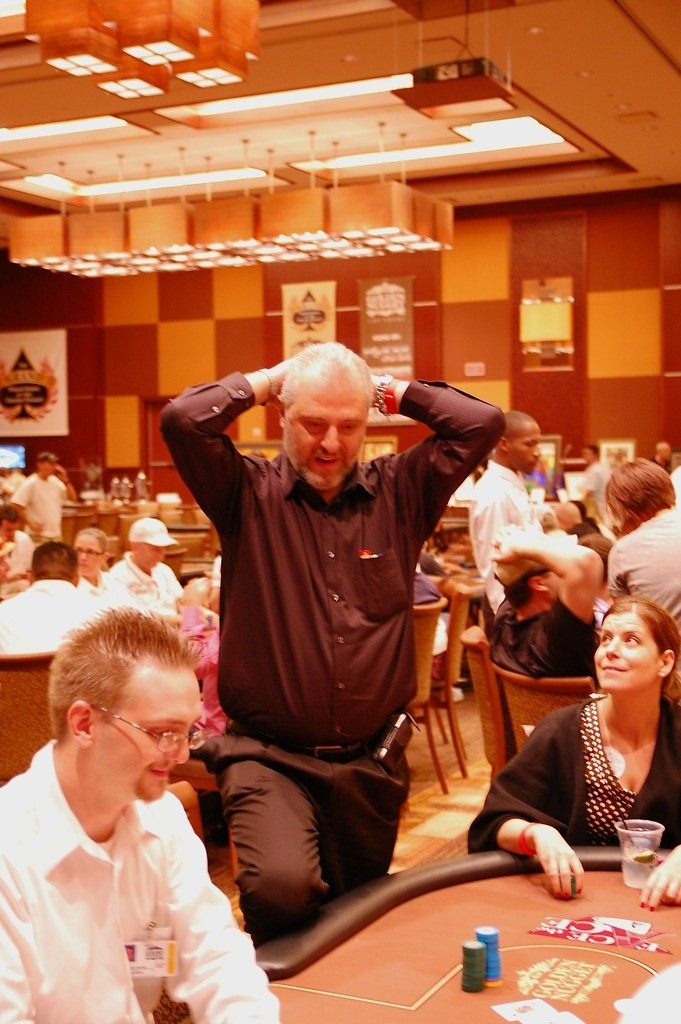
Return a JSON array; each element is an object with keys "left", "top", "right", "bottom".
[{"left": 614, "top": 819, "right": 665, "bottom": 889}]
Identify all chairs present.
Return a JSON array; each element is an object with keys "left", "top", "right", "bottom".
[
  {"left": 0, "top": 655, "right": 62, "bottom": 787},
  {"left": 60, "top": 500, "right": 223, "bottom": 577},
  {"left": 399, "top": 566, "right": 598, "bottom": 795}
]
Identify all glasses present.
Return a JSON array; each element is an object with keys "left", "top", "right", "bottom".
[
  {"left": 92, "top": 705, "right": 209, "bottom": 752},
  {"left": 76, "top": 547, "right": 105, "bottom": 557}
]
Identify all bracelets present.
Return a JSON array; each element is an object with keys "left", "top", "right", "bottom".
[
  {"left": 254, "top": 368, "right": 277, "bottom": 407},
  {"left": 65, "top": 481, "right": 71, "bottom": 485},
  {"left": 371, "top": 375, "right": 399, "bottom": 418},
  {"left": 518, "top": 822, "right": 539, "bottom": 856}
]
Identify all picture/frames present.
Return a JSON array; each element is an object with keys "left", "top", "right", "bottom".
[
  {"left": 599, "top": 439, "right": 637, "bottom": 472},
  {"left": 526, "top": 434, "right": 561, "bottom": 501}
]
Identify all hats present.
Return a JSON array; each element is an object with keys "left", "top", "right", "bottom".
[
  {"left": 38, "top": 452, "right": 59, "bottom": 462},
  {"left": 129, "top": 517, "right": 177, "bottom": 545},
  {"left": 493, "top": 531, "right": 578, "bottom": 588}
]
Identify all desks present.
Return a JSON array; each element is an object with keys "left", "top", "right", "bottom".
[{"left": 257, "top": 845, "right": 681, "bottom": 1024}]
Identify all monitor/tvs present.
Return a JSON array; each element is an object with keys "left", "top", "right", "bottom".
[{"left": 0, "top": 446, "right": 26, "bottom": 470}]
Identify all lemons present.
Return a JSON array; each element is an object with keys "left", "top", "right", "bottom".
[{"left": 632, "top": 851, "right": 657, "bottom": 863}]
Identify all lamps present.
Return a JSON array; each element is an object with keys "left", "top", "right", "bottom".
[
  {"left": 23, "top": 1, "right": 262, "bottom": 96},
  {"left": 8, "top": 120, "right": 452, "bottom": 279}
]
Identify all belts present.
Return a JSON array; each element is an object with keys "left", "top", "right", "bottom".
[{"left": 225, "top": 720, "right": 384, "bottom": 763}]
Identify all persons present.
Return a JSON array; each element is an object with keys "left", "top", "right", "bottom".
[
  {"left": 0, "top": 452, "right": 230, "bottom": 761},
  {"left": 0, "top": 605, "right": 281, "bottom": 1024},
  {"left": 125, "top": 946, "right": 134, "bottom": 961},
  {"left": 467, "top": 596, "right": 681, "bottom": 913},
  {"left": 159, "top": 342, "right": 507, "bottom": 948},
  {"left": 415, "top": 411, "right": 681, "bottom": 702}
]
